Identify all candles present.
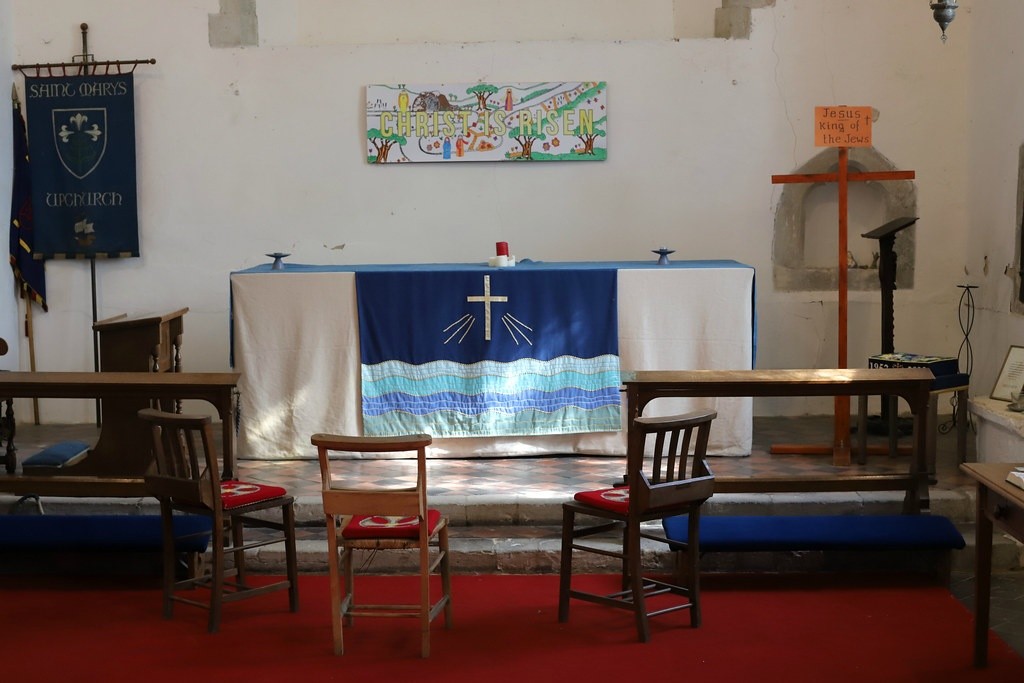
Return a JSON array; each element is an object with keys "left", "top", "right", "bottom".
[{"left": 495, "top": 242, "right": 509, "bottom": 257}]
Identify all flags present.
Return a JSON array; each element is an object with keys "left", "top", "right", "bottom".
[{"left": 10, "top": 100, "right": 49, "bottom": 312}]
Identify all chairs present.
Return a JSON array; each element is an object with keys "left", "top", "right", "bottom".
[
  {"left": 310, "top": 432, "right": 453, "bottom": 657},
  {"left": 140, "top": 408, "right": 299, "bottom": 635},
  {"left": 559, "top": 409, "right": 718, "bottom": 642}
]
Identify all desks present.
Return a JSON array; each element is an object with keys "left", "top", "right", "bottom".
[
  {"left": 0, "top": 369, "right": 240, "bottom": 547},
  {"left": 624, "top": 368, "right": 936, "bottom": 514},
  {"left": 961, "top": 463, "right": 1024, "bottom": 669},
  {"left": 232, "top": 260, "right": 760, "bottom": 457}
]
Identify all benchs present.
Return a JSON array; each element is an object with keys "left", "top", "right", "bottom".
[
  {"left": 22, "top": 442, "right": 91, "bottom": 477},
  {"left": 0, "top": 514, "right": 213, "bottom": 585},
  {"left": 661, "top": 512, "right": 964, "bottom": 582}
]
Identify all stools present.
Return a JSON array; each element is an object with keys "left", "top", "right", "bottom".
[{"left": 854, "top": 376, "right": 969, "bottom": 478}]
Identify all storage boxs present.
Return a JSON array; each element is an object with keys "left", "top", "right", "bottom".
[{"left": 867, "top": 353, "right": 958, "bottom": 377}]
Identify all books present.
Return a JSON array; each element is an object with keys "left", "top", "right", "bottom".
[{"left": 1005, "top": 471, "right": 1024, "bottom": 490}]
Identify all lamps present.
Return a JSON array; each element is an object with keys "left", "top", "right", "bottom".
[{"left": 929, "top": 0, "right": 959, "bottom": 41}]
